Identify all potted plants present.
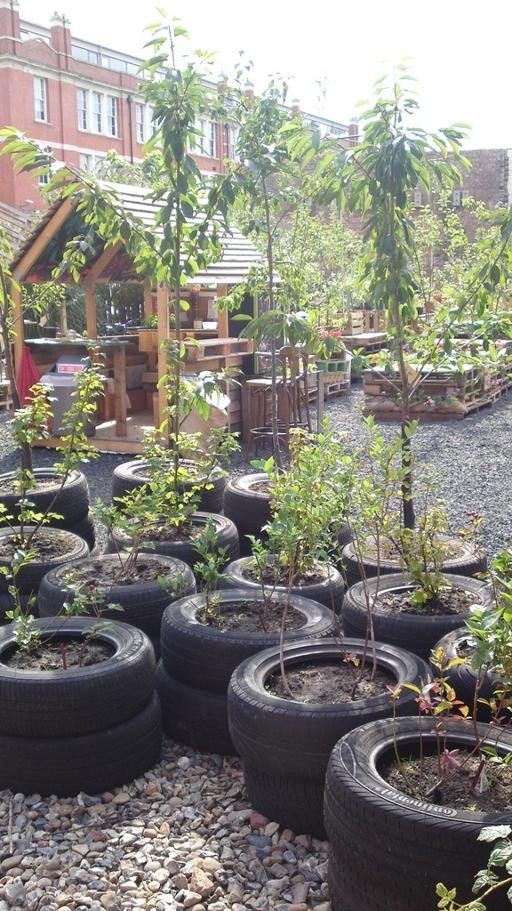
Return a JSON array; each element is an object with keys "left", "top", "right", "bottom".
[{"left": 0, "top": 12, "right": 511, "bottom": 911}]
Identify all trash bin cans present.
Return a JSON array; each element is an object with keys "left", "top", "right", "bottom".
[{"left": 36, "top": 355, "right": 95, "bottom": 439}]
